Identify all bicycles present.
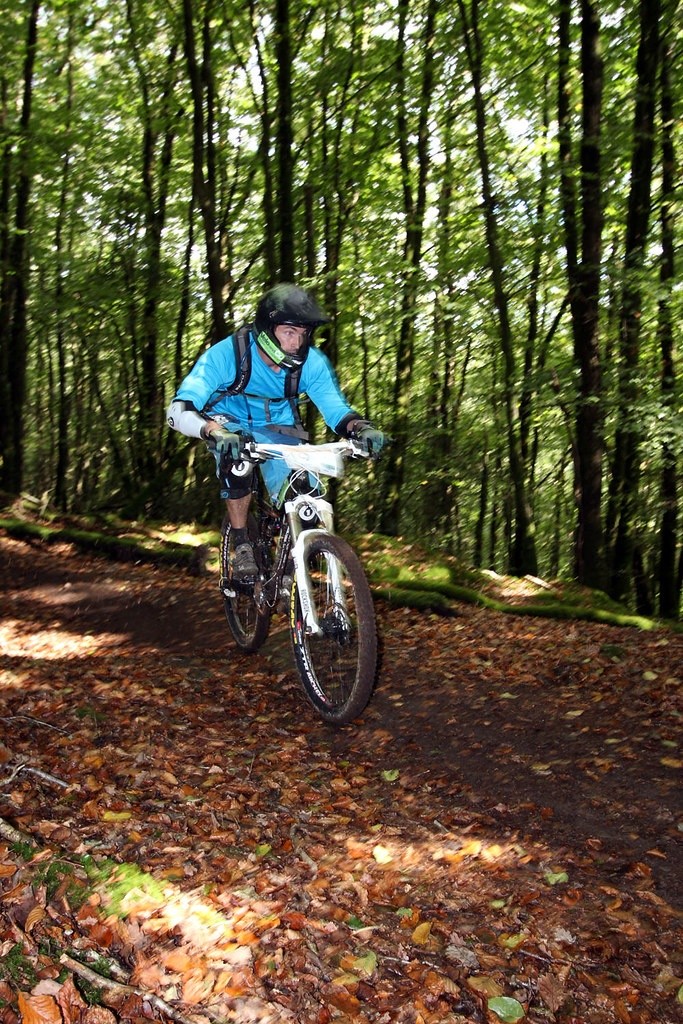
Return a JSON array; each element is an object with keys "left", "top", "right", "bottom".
[{"left": 208, "top": 438, "right": 378, "bottom": 728}]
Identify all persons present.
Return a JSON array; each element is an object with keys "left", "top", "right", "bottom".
[{"left": 165, "top": 284, "right": 391, "bottom": 575}]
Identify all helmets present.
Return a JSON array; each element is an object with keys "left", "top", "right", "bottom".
[{"left": 256, "top": 285, "right": 330, "bottom": 371}]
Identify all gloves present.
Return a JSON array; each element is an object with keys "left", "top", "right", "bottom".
[
  {"left": 334, "top": 411, "right": 385, "bottom": 456},
  {"left": 201, "top": 421, "right": 241, "bottom": 461}
]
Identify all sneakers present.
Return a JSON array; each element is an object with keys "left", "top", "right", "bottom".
[{"left": 231, "top": 543, "right": 259, "bottom": 575}]
